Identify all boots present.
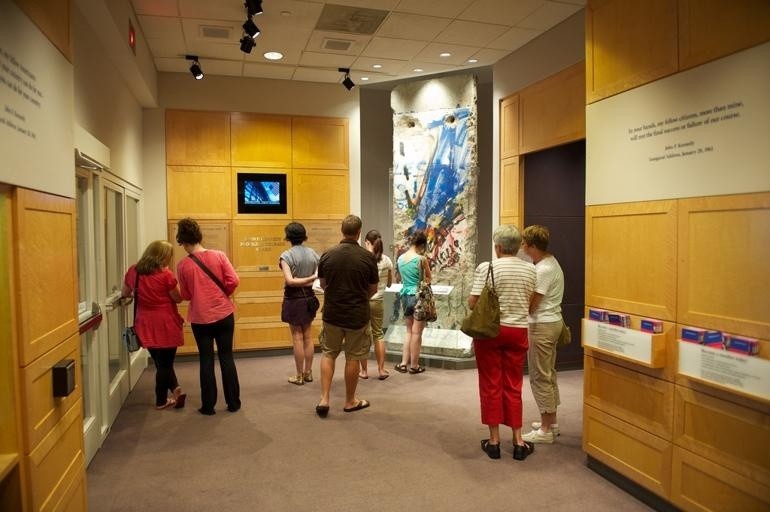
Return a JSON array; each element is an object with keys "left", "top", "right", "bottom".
[
  {"left": 302, "top": 369, "right": 312, "bottom": 381},
  {"left": 288, "top": 375, "right": 303, "bottom": 384}
]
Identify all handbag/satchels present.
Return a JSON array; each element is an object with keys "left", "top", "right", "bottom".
[
  {"left": 413, "top": 291, "right": 437, "bottom": 321},
  {"left": 307, "top": 297, "right": 319, "bottom": 311},
  {"left": 461, "top": 287, "right": 500, "bottom": 338},
  {"left": 558, "top": 324, "right": 571, "bottom": 345},
  {"left": 126, "top": 326, "right": 140, "bottom": 352}
]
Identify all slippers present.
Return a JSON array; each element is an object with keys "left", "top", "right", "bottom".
[
  {"left": 174, "top": 394, "right": 186, "bottom": 409},
  {"left": 344, "top": 400, "right": 369, "bottom": 412},
  {"left": 157, "top": 399, "right": 175, "bottom": 409},
  {"left": 379, "top": 370, "right": 389, "bottom": 379},
  {"left": 316, "top": 406, "right": 329, "bottom": 413}
]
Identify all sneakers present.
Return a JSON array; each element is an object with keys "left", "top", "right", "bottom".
[
  {"left": 521, "top": 430, "right": 554, "bottom": 444},
  {"left": 532, "top": 421, "right": 559, "bottom": 436}
]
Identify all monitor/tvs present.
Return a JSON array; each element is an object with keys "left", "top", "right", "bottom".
[{"left": 237, "top": 172, "right": 287, "bottom": 214}]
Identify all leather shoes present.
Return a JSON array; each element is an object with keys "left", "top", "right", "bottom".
[
  {"left": 482, "top": 438, "right": 500, "bottom": 458},
  {"left": 513, "top": 441, "right": 534, "bottom": 460}
]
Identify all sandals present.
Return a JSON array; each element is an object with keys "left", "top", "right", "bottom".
[
  {"left": 395, "top": 364, "right": 407, "bottom": 373},
  {"left": 409, "top": 365, "right": 425, "bottom": 374}
]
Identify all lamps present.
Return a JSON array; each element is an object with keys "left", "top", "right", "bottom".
[
  {"left": 184, "top": 54, "right": 205, "bottom": 80},
  {"left": 337, "top": 67, "right": 356, "bottom": 91},
  {"left": 239, "top": 0, "right": 264, "bottom": 54}
]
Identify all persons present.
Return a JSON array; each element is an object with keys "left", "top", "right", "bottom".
[
  {"left": 176, "top": 218, "right": 241, "bottom": 414},
  {"left": 316, "top": 214, "right": 379, "bottom": 412},
  {"left": 122, "top": 241, "right": 186, "bottom": 410},
  {"left": 358, "top": 230, "right": 393, "bottom": 380},
  {"left": 521, "top": 224, "right": 565, "bottom": 445},
  {"left": 277, "top": 221, "right": 321, "bottom": 385},
  {"left": 468, "top": 225, "right": 534, "bottom": 460},
  {"left": 394, "top": 231, "right": 432, "bottom": 374}
]
